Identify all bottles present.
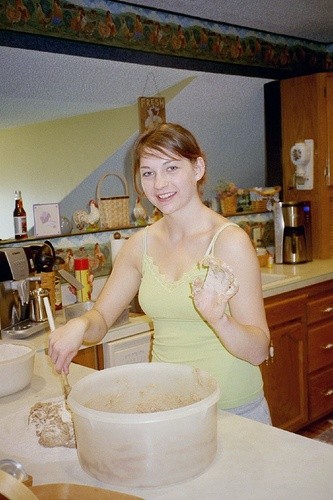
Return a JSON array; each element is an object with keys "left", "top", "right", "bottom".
[
  {"left": 254, "top": 240, "right": 267, "bottom": 267},
  {"left": 13, "top": 191, "right": 28, "bottom": 240}
]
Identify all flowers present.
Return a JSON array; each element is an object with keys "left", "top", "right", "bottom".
[{"left": 216, "top": 177, "right": 244, "bottom": 199}]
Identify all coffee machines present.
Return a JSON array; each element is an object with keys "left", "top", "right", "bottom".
[{"left": 0, "top": 249, "right": 52, "bottom": 339}]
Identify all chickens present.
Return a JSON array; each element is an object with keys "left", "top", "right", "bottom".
[
  {"left": 133, "top": 195, "right": 146, "bottom": 220},
  {"left": 72, "top": 200, "right": 101, "bottom": 230}
]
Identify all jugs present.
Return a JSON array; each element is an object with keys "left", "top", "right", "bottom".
[{"left": 282, "top": 201, "right": 308, "bottom": 263}]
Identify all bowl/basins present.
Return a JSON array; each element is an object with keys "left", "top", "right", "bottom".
[
  {"left": 21, "top": 475, "right": 33, "bottom": 487},
  {"left": 67, "top": 362, "right": 220, "bottom": 487},
  {"left": 0, "top": 339, "right": 35, "bottom": 397}
]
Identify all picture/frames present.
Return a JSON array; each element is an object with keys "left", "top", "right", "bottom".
[
  {"left": 33, "top": 203, "right": 61, "bottom": 237},
  {"left": 220, "top": 197, "right": 237, "bottom": 215}
]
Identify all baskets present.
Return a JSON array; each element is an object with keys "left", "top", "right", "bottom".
[{"left": 95, "top": 171, "right": 129, "bottom": 228}]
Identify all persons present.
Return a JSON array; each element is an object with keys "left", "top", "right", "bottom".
[{"left": 47, "top": 122, "right": 272, "bottom": 427}]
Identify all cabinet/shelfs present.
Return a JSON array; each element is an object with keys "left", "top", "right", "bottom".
[{"left": 0, "top": 73, "right": 333, "bottom": 500}]
